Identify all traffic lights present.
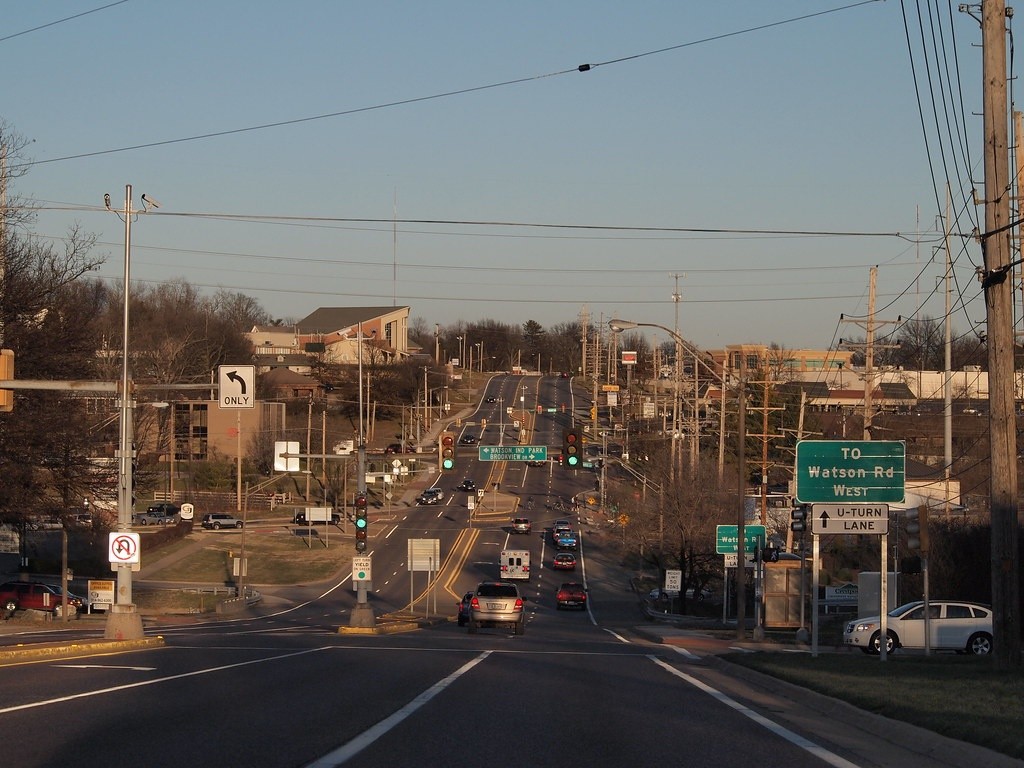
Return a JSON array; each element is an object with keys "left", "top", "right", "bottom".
[
  {"left": 563, "top": 427, "right": 583, "bottom": 471},
  {"left": 790, "top": 496, "right": 807, "bottom": 533},
  {"left": 439, "top": 429, "right": 456, "bottom": 474},
  {"left": 354, "top": 494, "right": 366, "bottom": 552},
  {"left": 762, "top": 547, "right": 780, "bottom": 563}
]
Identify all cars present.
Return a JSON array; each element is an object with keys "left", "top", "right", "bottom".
[
  {"left": 200, "top": 513, "right": 242, "bottom": 530},
  {"left": 297, "top": 511, "right": 340, "bottom": 526},
  {"left": 420, "top": 488, "right": 444, "bottom": 506},
  {"left": 501, "top": 550, "right": 530, "bottom": 579},
  {"left": 460, "top": 478, "right": 473, "bottom": 493},
  {"left": 0, "top": 582, "right": 93, "bottom": 614},
  {"left": 469, "top": 582, "right": 525, "bottom": 634},
  {"left": 462, "top": 433, "right": 475, "bottom": 445},
  {"left": 554, "top": 582, "right": 588, "bottom": 610},
  {"left": 485, "top": 395, "right": 497, "bottom": 405},
  {"left": 841, "top": 600, "right": 994, "bottom": 656},
  {"left": 139, "top": 511, "right": 176, "bottom": 526},
  {"left": 552, "top": 519, "right": 578, "bottom": 549},
  {"left": 148, "top": 504, "right": 179, "bottom": 516},
  {"left": 511, "top": 517, "right": 530, "bottom": 536},
  {"left": 552, "top": 553, "right": 575, "bottom": 571}
]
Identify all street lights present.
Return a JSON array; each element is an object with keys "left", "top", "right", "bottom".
[
  {"left": 101, "top": 184, "right": 159, "bottom": 617},
  {"left": 605, "top": 315, "right": 751, "bottom": 644}
]
